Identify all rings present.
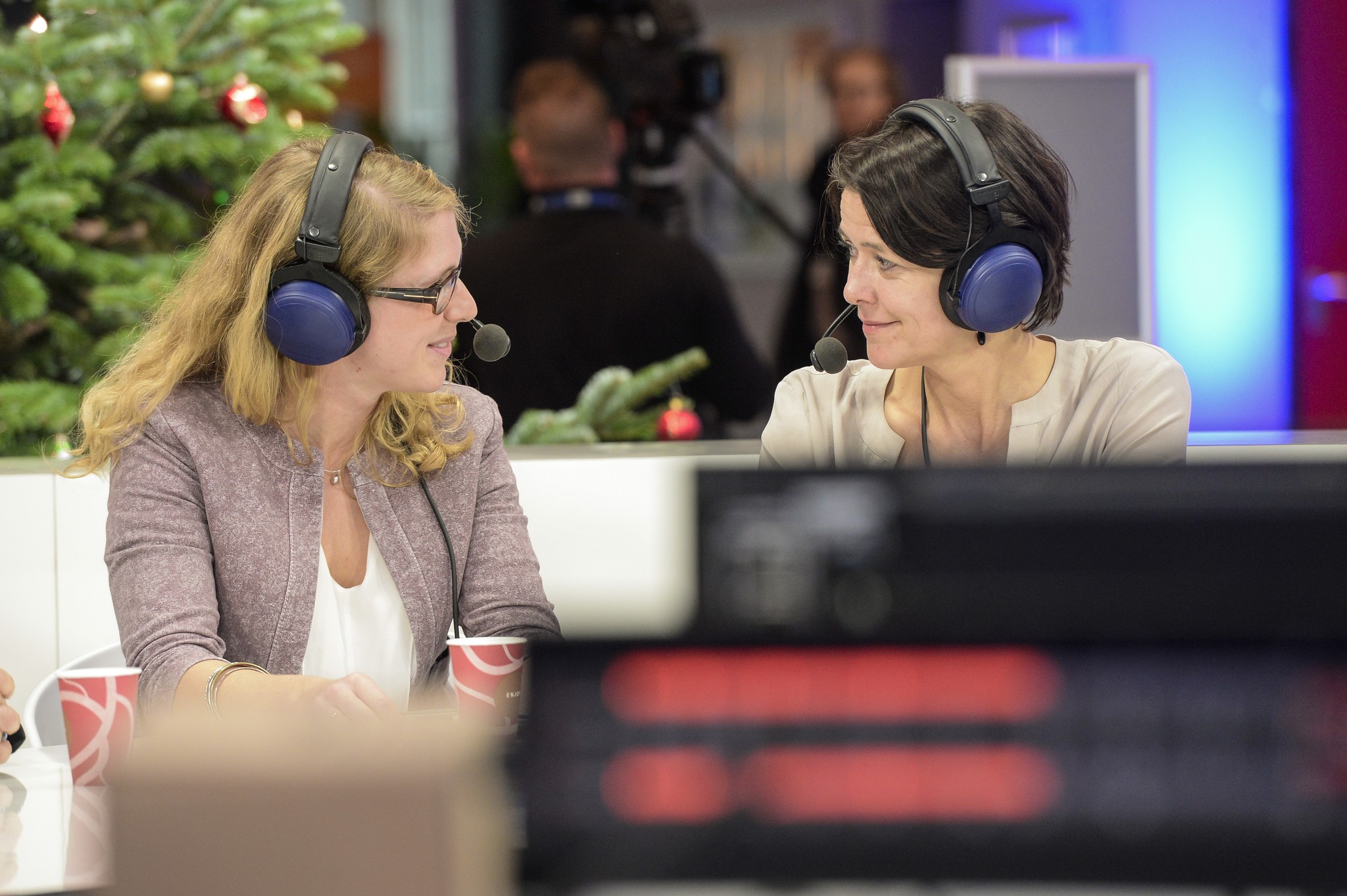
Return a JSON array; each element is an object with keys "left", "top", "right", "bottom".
[{"left": 2, "top": 732, "right": 8, "bottom": 741}]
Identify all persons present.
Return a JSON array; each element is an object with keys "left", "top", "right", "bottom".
[
  {"left": 757, "top": 96, "right": 1192, "bottom": 471},
  {"left": 68, "top": 134, "right": 566, "bottom": 731},
  {"left": 774, "top": 49, "right": 913, "bottom": 402},
  {"left": 0, "top": 667, "right": 20, "bottom": 764},
  {"left": 445, "top": 59, "right": 781, "bottom": 442}
]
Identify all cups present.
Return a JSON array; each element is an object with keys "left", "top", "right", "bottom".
[
  {"left": 56, "top": 666, "right": 143, "bottom": 787},
  {"left": 445, "top": 636, "right": 528, "bottom": 736}
]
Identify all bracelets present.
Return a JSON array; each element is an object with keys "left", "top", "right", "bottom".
[{"left": 205, "top": 660, "right": 271, "bottom": 711}]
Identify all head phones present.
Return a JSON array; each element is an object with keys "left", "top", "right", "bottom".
[
  {"left": 263, "top": 128, "right": 374, "bottom": 364},
  {"left": 890, "top": 99, "right": 1046, "bottom": 344}
]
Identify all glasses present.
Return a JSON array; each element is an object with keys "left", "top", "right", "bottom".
[{"left": 356, "top": 253, "right": 466, "bottom": 315}]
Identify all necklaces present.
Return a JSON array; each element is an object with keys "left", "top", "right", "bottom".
[{"left": 325, "top": 459, "right": 355, "bottom": 488}]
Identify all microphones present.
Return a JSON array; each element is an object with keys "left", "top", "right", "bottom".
[
  {"left": 810, "top": 304, "right": 857, "bottom": 374},
  {"left": 469, "top": 319, "right": 511, "bottom": 362}
]
